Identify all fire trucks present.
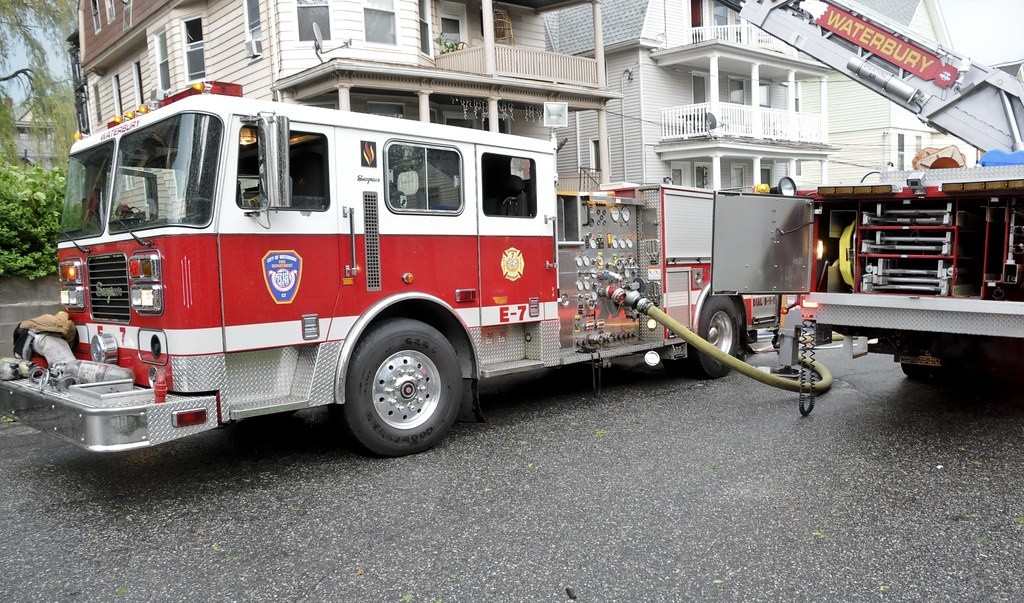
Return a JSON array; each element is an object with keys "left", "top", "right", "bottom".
[
  {"left": 712, "top": 0, "right": 1023, "bottom": 405},
  {"left": 1, "top": 83, "right": 824, "bottom": 460}
]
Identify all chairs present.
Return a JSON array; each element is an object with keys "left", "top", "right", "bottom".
[{"left": 495, "top": 13, "right": 516, "bottom": 45}]
